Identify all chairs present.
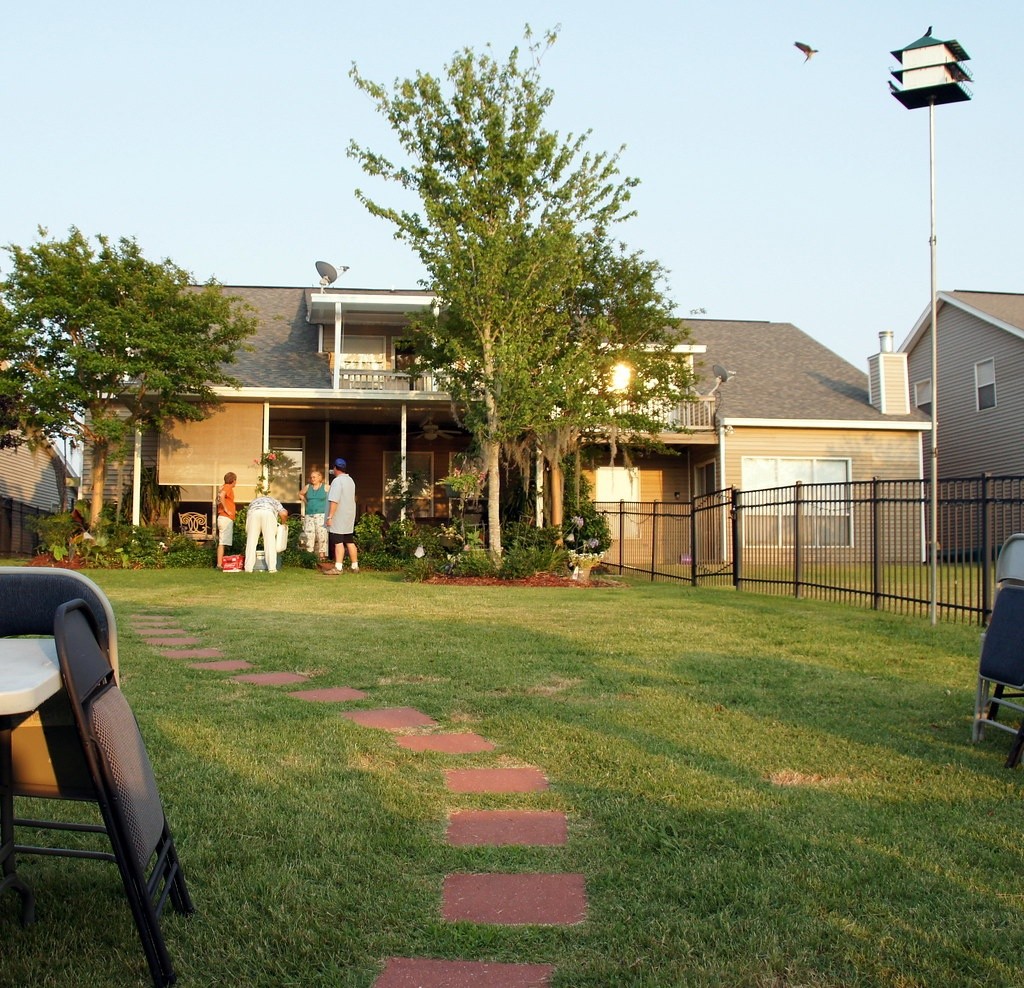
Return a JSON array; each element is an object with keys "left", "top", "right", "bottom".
[
  {"left": 178, "top": 512, "right": 214, "bottom": 545},
  {"left": 54, "top": 599, "right": 196, "bottom": 988},
  {"left": 0, "top": 565, "right": 120, "bottom": 861},
  {"left": 972, "top": 532, "right": 1024, "bottom": 744}
]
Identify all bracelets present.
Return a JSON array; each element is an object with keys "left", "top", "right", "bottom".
[{"left": 326, "top": 516, "right": 333, "bottom": 520}]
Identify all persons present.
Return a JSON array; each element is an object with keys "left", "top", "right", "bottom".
[
  {"left": 298, "top": 472, "right": 331, "bottom": 562},
  {"left": 216, "top": 472, "right": 237, "bottom": 570},
  {"left": 323, "top": 459, "right": 360, "bottom": 575},
  {"left": 244, "top": 496, "right": 289, "bottom": 573}
]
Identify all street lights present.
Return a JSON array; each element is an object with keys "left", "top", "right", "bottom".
[{"left": 887, "top": 26, "right": 974, "bottom": 627}]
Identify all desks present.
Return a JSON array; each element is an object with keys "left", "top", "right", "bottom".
[{"left": 0, "top": 637, "right": 63, "bottom": 931}]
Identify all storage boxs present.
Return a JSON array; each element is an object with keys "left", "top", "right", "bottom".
[{"left": 254, "top": 551, "right": 282, "bottom": 570}]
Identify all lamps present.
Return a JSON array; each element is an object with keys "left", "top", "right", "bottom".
[{"left": 424, "top": 429, "right": 437, "bottom": 439}]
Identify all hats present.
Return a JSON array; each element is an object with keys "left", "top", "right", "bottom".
[{"left": 335, "top": 458, "right": 346, "bottom": 470}]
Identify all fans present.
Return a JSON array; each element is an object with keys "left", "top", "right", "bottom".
[{"left": 408, "top": 416, "right": 462, "bottom": 440}]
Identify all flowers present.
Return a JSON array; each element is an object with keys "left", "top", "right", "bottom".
[
  {"left": 569, "top": 550, "right": 605, "bottom": 570},
  {"left": 436, "top": 523, "right": 464, "bottom": 542}
]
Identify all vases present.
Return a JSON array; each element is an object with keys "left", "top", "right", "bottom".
[
  {"left": 576, "top": 568, "right": 591, "bottom": 579},
  {"left": 439, "top": 536, "right": 453, "bottom": 546}
]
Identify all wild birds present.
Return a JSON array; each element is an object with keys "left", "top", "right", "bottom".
[{"left": 794, "top": 42, "right": 818, "bottom": 63}]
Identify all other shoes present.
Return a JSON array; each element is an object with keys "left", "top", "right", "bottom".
[
  {"left": 267, "top": 568, "right": 278, "bottom": 573},
  {"left": 244, "top": 567, "right": 254, "bottom": 573},
  {"left": 347, "top": 567, "right": 361, "bottom": 573},
  {"left": 324, "top": 566, "right": 344, "bottom": 574}
]
